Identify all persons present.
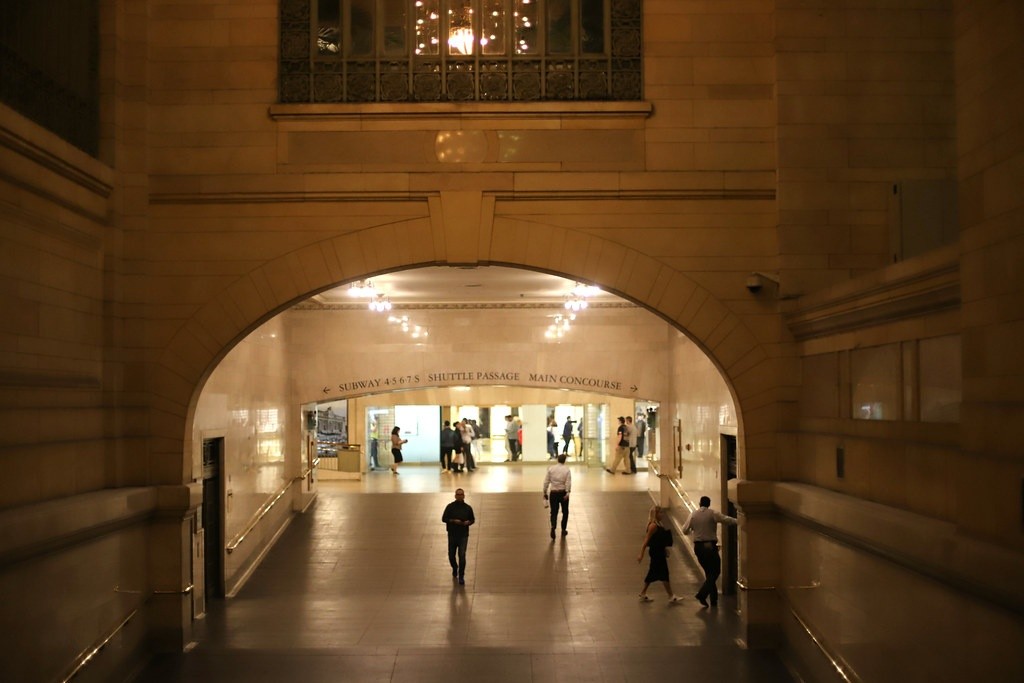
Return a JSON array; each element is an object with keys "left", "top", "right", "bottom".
[
  {"left": 389, "top": 425, "right": 408, "bottom": 475},
  {"left": 636, "top": 504, "right": 685, "bottom": 602},
  {"left": 370, "top": 414, "right": 381, "bottom": 468},
  {"left": 680, "top": 495, "right": 737, "bottom": 608},
  {"left": 439, "top": 416, "right": 483, "bottom": 472},
  {"left": 543, "top": 454, "right": 571, "bottom": 539},
  {"left": 502, "top": 414, "right": 524, "bottom": 462},
  {"left": 441, "top": 490, "right": 475, "bottom": 586},
  {"left": 606, "top": 412, "right": 646, "bottom": 475},
  {"left": 546, "top": 416, "right": 583, "bottom": 460}
]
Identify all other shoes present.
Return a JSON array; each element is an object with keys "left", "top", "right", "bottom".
[
  {"left": 607, "top": 469, "right": 615, "bottom": 475},
  {"left": 392, "top": 468, "right": 399, "bottom": 474},
  {"left": 442, "top": 467, "right": 477, "bottom": 474},
  {"left": 622, "top": 471, "right": 631, "bottom": 475},
  {"left": 562, "top": 531, "right": 568, "bottom": 535},
  {"left": 549, "top": 454, "right": 571, "bottom": 459},
  {"left": 550, "top": 527, "right": 556, "bottom": 539},
  {"left": 453, "top": 565, "right": 458, "bottom": 577},
  {"left": 504, "top": 458, "right": 517, "bottom": 462},
  {"left": 459, "top": 576, "right": 464, "bottom": 585}
]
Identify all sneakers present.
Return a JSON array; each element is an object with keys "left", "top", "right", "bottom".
[
  {"left": 695, "top": 593, "right": 710, "bottom": 607},
  {"left": 639, "top": 594, "right": 654, "bottom": 602},
  {"left": 668, "top": 594, "right": 685, "bottom": 603},
  {"left": 711, "top": 599, "right": 717, "bottom": 605}
]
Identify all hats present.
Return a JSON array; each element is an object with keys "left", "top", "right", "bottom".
[{"left": 617, "top": 417, "right": 625, "bottom": 422}]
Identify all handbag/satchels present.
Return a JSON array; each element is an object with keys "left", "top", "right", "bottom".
[{"left": 647, "top": 529, "right": 673, "bottom": 548}]
